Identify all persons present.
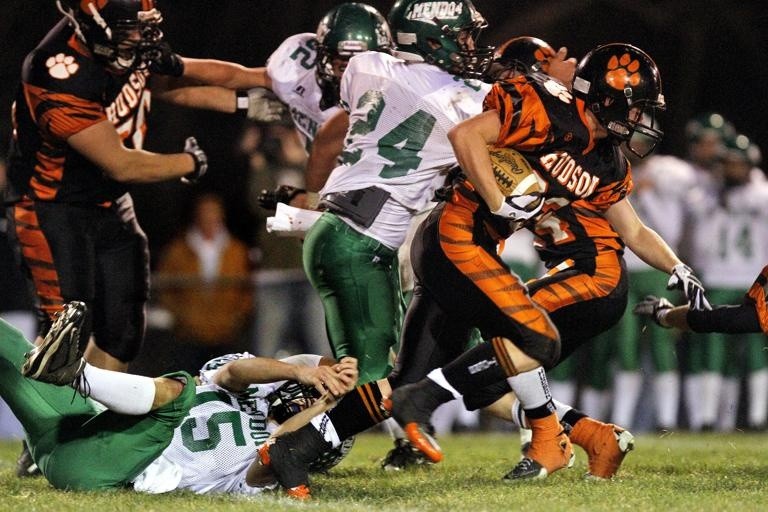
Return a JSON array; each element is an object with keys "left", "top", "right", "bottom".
[{"left": 1, "top": 0, "right": 768, "bottom": 499}]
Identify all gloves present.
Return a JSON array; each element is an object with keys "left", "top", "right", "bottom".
[
  {"left": 667, "top": 263, "right": 712, "bottom": 312},
  {"left": 256, "top": 184, "right": 306, "bottom": 212},
  {"left": 491, "top": 195, "right": 547, "bottom": 222},
  {"left": 181, "top": 137, "right": 210, "bottom": 183},
  {"left": 235, "top": 86, "right": 287, "bottom": 124},
  {"left": 633, "top": 295, "right": 674, "bottom": 329}
]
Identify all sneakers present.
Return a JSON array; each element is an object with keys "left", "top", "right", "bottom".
[
  {"left": 21, "top": 300, "right": 93, "bottom": 385},
  {"left": 379, "top": 391, "right": 445, "bottom": 472},
  {"left": 255, "top": 437, "right": 314, "bottom": 503},
  {"left": 501, "top": 423, "right": 635, "bottom": 483},
  {"left": 18, "top": 440, "right": 44, "bottom": 478}
]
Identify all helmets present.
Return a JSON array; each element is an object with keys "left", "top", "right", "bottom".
[
  {"left": 265, "top": 381, "right": 357, "bottom": 474},
  {"left": 484, "top": 36, "right": 557, "bottom": 82},
  {"left": 316, "top": 2, "right": 393, "bottom": 111},
  {"left": 390, "top": 0, "right": 495, "bottom": 77},
  {"left": 59, "top": 0, "right": 166, "bottom": 70},
  {"left": 572, "top": 43, "right": 666, "bottom": 160},
  {"left": 681, "top": 115, "right": 762, "bottom": 188}
]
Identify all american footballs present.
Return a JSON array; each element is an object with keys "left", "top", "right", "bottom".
[{"left": 486, "top": 148, "right": 545, "bottom": 212}]
